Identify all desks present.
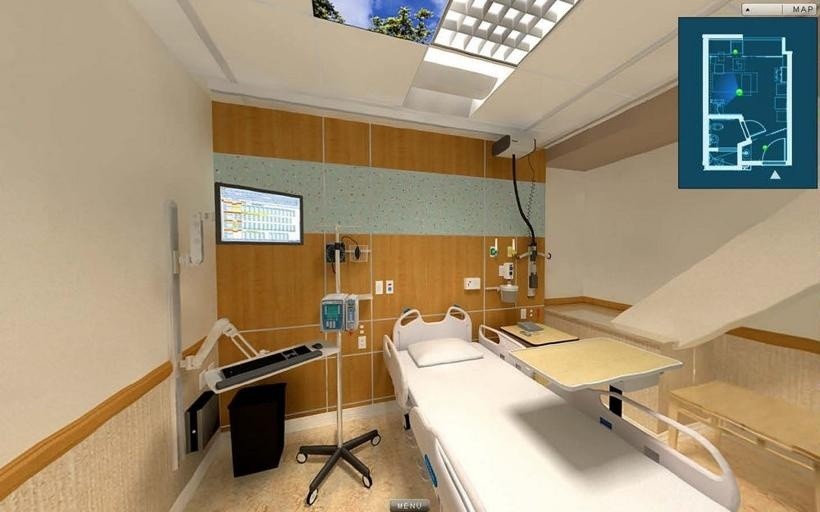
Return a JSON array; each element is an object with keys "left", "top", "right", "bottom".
[
  {"left": 668, "top": 380, "right": 820, "bottom": 511},
  {"left": 500, "top": 322, "right": 579, "bottom": 347},
  {"left": 509, "top": 335, "right": 684, "bottom": 391}
]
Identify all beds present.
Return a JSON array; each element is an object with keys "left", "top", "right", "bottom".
[{"left": 382, "top": 306, "right": 740, "bottom": 512}]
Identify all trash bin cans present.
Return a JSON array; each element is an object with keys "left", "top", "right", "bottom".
[{"left": 228, "top": 383, "right": 287, "bottom": 477}]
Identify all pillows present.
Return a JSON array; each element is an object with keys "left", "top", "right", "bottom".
[{"left": 408, "top": 338, "right": 484, "bottom": 367}]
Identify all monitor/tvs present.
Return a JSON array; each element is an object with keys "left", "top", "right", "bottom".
[{"left": 214, "top": 182, "right": 305, "bottom": 244}]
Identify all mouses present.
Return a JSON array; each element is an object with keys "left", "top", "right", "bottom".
[{"left": 312, "top": 342, "right": 323, "bottom": 350}]
